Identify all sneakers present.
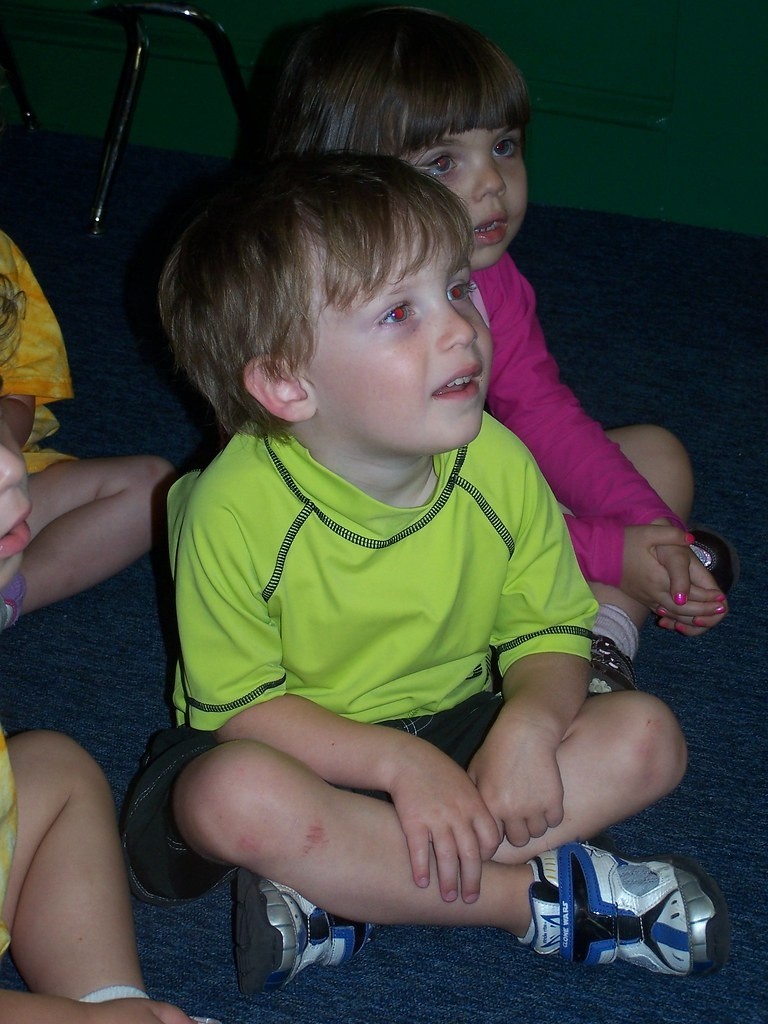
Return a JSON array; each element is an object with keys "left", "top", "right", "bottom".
[
  {"left": 235, "top": 867, "right": 375, "bottom": 995},
  {"left": 589, "top": 634, "right": 638, "bottom": 695},
  {"left": 528, "top": 838, "right": 730, "bottom": 977},
  {"left": 688, "top": 525, "right": 740, "bottom": 595}
]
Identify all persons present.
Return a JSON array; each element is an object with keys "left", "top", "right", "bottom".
[
  {"left": 166, "top": 153, "right": 728, "bottom": 993},
  {"left": 1, "top": 228, "right": 174, "bottom": 629},
  {"left": 268, "top": 11, "right": 736, "bottom": 693},
  {"left": 1, "top": 265, "right": 223, "bottom": 1024}
]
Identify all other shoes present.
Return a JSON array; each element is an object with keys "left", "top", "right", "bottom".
[{"left": 0, "top": 574, "right": 26, "bottom": 633}]
